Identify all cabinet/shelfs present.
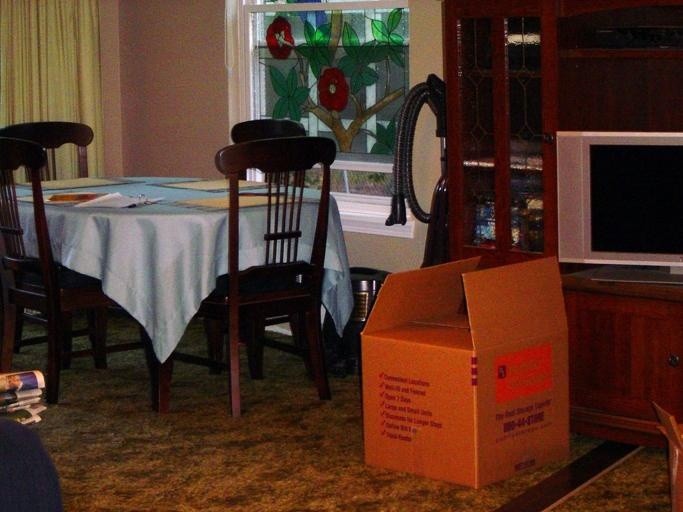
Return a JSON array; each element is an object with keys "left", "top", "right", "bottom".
[{"left": 441, "top": 0, "right": 683, "bottom": 451}]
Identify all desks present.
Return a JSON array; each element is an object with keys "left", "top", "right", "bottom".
[{"left": 2, "top": 177, "right": 340, "bottom": 412}]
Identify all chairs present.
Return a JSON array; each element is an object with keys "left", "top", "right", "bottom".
[
  {"left": 0, "top": 121, "right": 107, "bottom": 369},
  {"left": 0, "top": 136, "right": 158, "bottom": 410},
  {"left": 175, "top": 137, "right": 336, "bottom": 415},
  {"left": 204, "top": 119, "right": 306, "bottom": 380}
]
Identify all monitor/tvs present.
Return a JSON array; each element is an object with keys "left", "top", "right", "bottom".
[{"left": 557, "top": 131, "right": 683, "bottom": 283}]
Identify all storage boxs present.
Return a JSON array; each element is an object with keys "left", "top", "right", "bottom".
[{"left": 361, "top": 255, "right": 570, "bottom": 490}]
